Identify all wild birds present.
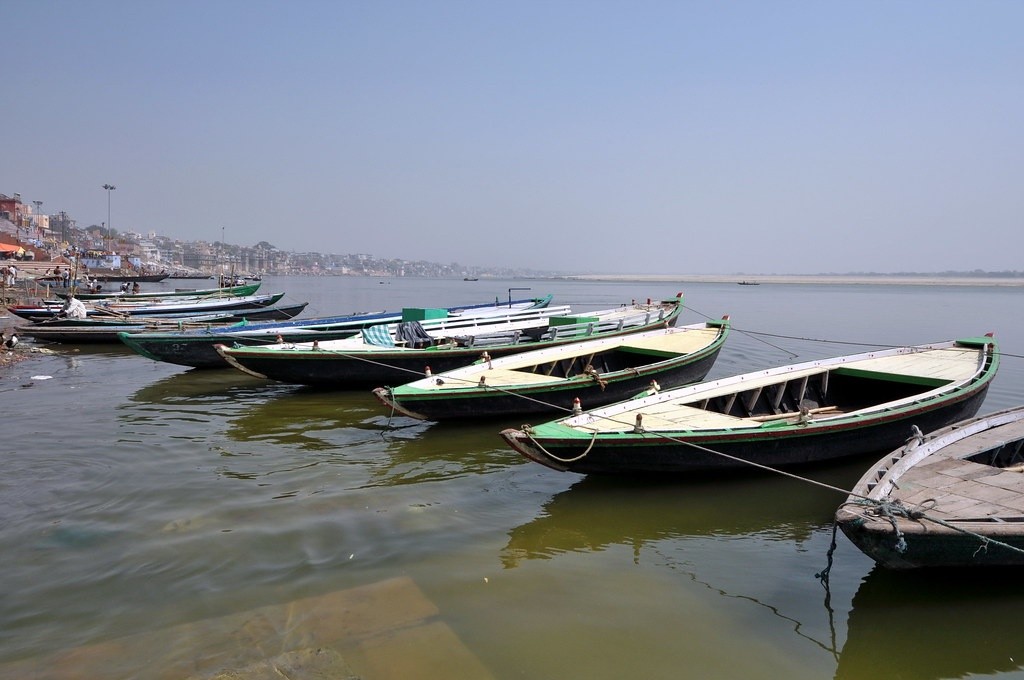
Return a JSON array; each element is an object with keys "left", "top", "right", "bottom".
[{"left": 0, "top": 332, "right": 19, "bottom": 351}]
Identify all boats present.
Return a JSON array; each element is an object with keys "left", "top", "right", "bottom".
[
  {"left": 837, "top": 406, "right": 1024, "bottom": 576},
  {"left": 82, "top": 273, "right": 171, "bottom": 282},
  {"left": 115, "top": 293, "right": 547, "bottom": 369},
  {"left": 212, "top": 291, "right": 685, "bottom": 387},
  {"left": 217, "top": 271, "right": 262, "bottom": 286},
  {"left": 34, "top": 278, "right": 79, "bottom": 287},
  {"left": 369, "top": 314, "right": 728, "bottom": 421},
  {"left": 6, "top": 280, "right": 312, "bottom": 346},
  {"left": 464, "top": 277, "right": 478, "bottom": 282},
  {"left": 501, "top": 331, "right": 1003, "bottom": 473}
]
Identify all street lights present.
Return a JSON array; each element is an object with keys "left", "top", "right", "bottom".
[{"left": 103, "top": 184, "right": 116, "bottom": 252}]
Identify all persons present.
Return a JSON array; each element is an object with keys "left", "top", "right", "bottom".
[
  {"left": 120, "top": 280, "right": 141, "bottom": 294},
  {"left": 82, "top": 271, "right": 108, "bottom": 294},
  {"left": 0, "top": 265, "right": 18, "bottom": 288},
  {"left": 43, "top": 266, "right": 77, "bottom": 288},
  {"left": 54, "top": 292, "right": 86, "bottom": 319},
  {"left": 29, "top": 238, "right": 113, "bottom": 258}
]
